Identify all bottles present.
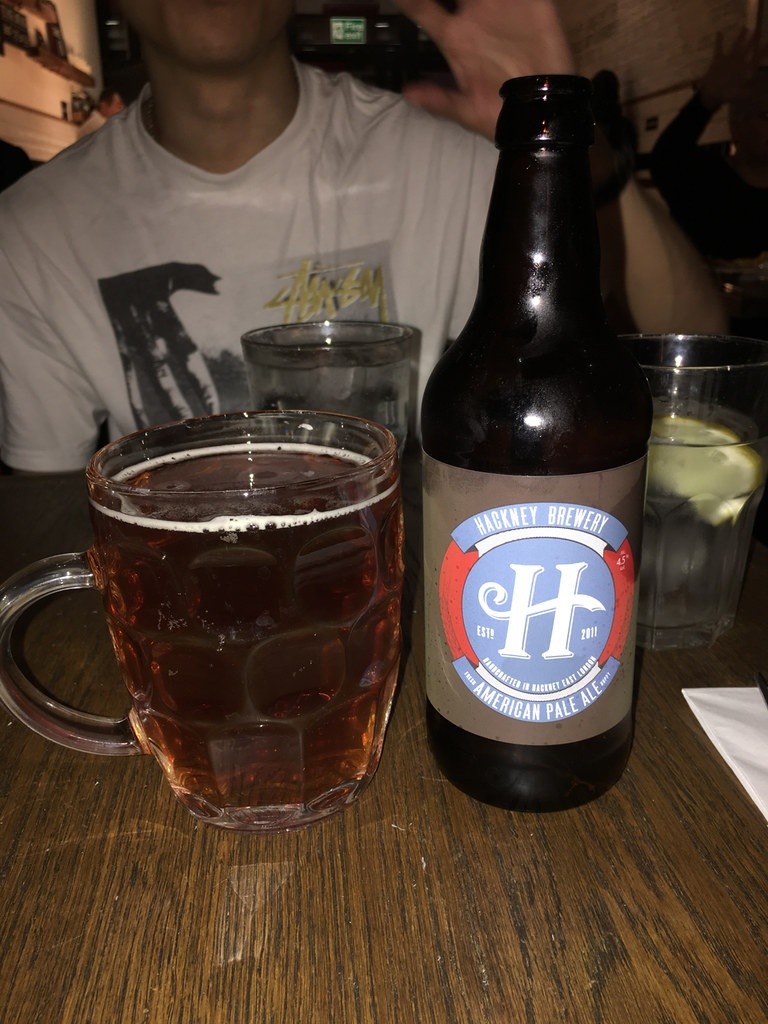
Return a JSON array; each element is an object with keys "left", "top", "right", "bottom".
[{"left": 418, "top": 73, "right": 653, "bottom": 813}]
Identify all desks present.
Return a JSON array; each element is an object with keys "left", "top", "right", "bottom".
[{"left": 1, "top": 471, "right": 767, "bottom": 1024}]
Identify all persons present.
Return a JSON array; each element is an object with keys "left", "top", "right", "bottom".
[{"left": 0, "top": 0, "right": 728, "bottom": 475}]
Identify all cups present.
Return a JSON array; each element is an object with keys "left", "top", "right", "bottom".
[
  {"left": 0, "top": 401, "right": 407, "bottom": 831},
  {"left": 624, "top": 332, "right": 768, "bottom": 652},
  {"left": 242, "top": 320, "right": 413, "bottom": 462}
]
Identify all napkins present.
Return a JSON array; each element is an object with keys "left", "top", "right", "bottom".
[{"left": 682, "top": 684, "right": 767, "bottom": 824}]
